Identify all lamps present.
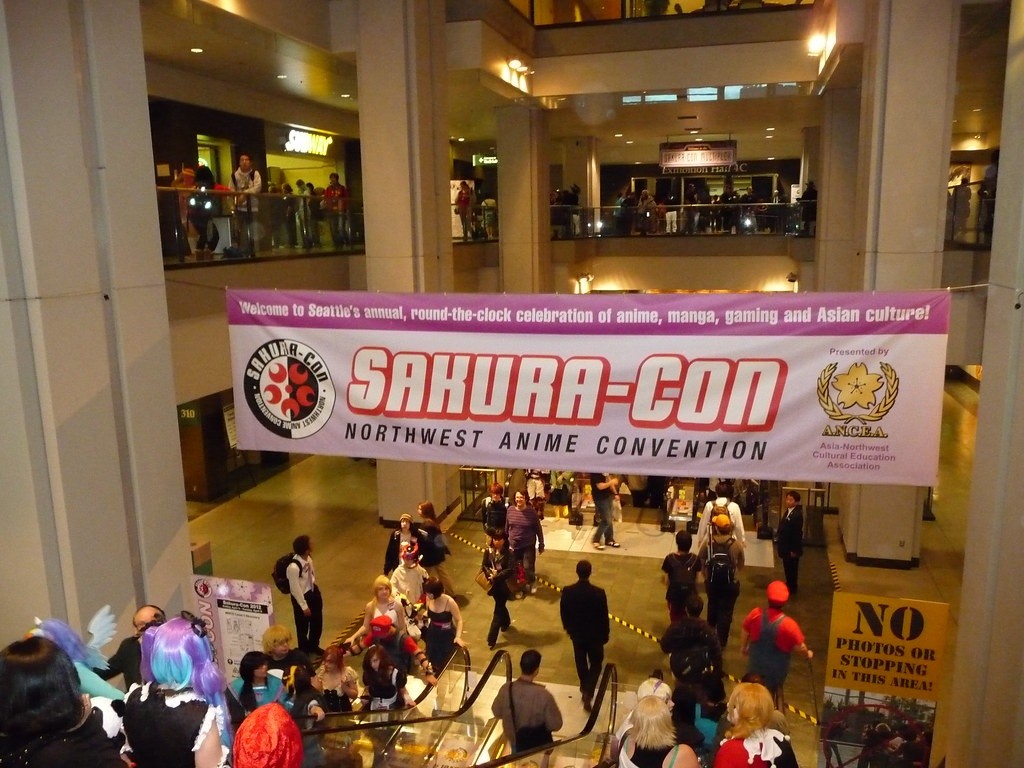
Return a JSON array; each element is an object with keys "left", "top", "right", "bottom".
[
  {"left": 576, "top": 271, "right": 595, "bottom": 282},
  {"left": 506, "top": 55, "right": 535, "bottom": 76},
  {"left": 808, "top": 48, "right": 822, "bottom": 57},
  {"left": 786, "top": 271, "right": 797, "bottom": 281}
]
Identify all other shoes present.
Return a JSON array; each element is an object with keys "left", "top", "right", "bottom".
[
  {"left": 606, "top": 540, "right": 620, "bottom": 547},
  {"left": 593, "top": 541, "right": 605, "bottom": 550}
]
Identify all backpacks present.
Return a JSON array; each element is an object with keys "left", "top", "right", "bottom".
[
  {"left": 670, "top": 629, "right": 715, "bottom": 682},
  {"left": 666, "top": 553, "right": 697, "bottom": 594},
  {"left": 710, "top": 500, "right": 731, "bottom": 523},
  {"left": 271, "top": 552, "right": 311, "bottom": 594},
  {"left": 705, "top": 537, "right": 740, "bottom": 597}
]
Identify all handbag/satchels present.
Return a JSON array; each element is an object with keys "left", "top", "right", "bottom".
[
  {"left": 505, "top": 574, "right": 528, "bottom": 600},
  {"left": 474, "top": 568, "right": 492, "bottom": 590},
  {"left": 508, "top": 682, "right": 554, "bottom": 755}
]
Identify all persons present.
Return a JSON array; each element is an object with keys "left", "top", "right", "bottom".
[
  {"left": 823, "top": 706, "right": 930, "bottom": 768},
  {"left": 950, "top": 174, "right": 995, "bottom": 249},
  {"left": 172, "top": 153, "right": 351, "bottom": 261},
  {"left": 550, "top": 180, "right": 817, "bottom": 237},
  {"left": 456, "top": 181, "right": 497, "bottom": 242},
  {"left": 0, "top": 469, "right": 813, "bottom": 768}
]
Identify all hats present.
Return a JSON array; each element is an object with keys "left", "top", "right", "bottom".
[
  {"left": 711, "top": 514, "right": 731, "bottom": 528},
  {"left": 400, "top": 513, "right": 415, "bottom": 523},
  {"left": 570, "top": 183, "right": 581, "bottom": 194},
  {"left": 767, "top": 580, "right": 789, "bottom": 606},
  {"left": 370, "top": 615, "right": 392, "bottom": 638}
]
